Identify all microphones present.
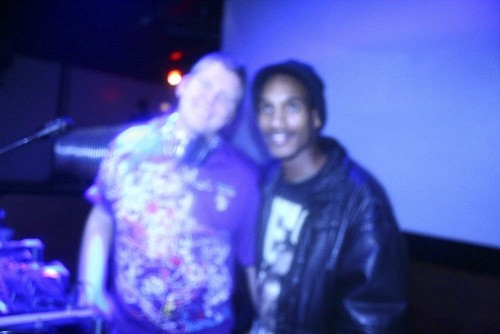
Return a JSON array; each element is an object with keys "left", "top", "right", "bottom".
[{"left": 38, "top": 118, "right": 73, "bottom": 139}]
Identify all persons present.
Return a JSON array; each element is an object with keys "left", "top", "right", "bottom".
[
  {"left": 75, "top": 53, "right": 264, "bottom": 334},
  {"left": 243, "top": 58, "right": 413, "bottom": 334}
]
